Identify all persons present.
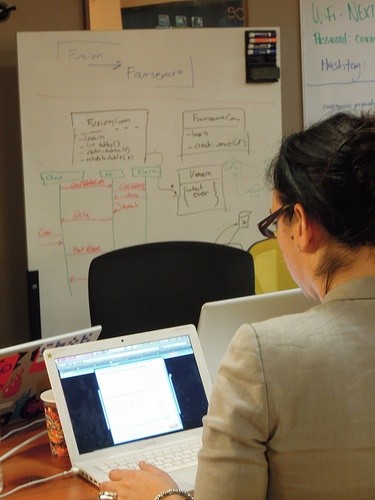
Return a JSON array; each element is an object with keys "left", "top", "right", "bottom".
[{"left": 96, "top": 112, "right": 375, "bottom": 500}]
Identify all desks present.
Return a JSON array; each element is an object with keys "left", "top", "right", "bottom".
[{"left": 0, "top": 416, "right": 100, "bottom": 500}]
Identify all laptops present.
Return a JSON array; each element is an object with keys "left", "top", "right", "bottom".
[
  {"left": 0, "top": 325, "right": 101, "bottom": 426},
  {"left": 196, "top": 287, "right": 322, "bottom": 385},
  {"left": 43, "top": 324, "right": 214, "bottom": 500}
]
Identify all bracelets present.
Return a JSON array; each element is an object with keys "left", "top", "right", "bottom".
[{"left": 153, "top": 488, "right": 194, "bottom": 500}]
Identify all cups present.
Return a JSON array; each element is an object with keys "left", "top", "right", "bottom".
[{"left": 39, "top": 388, "right": 69, "bottom": 458}]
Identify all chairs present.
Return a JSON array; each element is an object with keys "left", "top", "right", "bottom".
[{"left": 88, "top": 241, "right": 255, "bottom": 340}]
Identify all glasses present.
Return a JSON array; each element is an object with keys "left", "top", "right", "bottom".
[{"left": 258, "top": 205, "right": 296, "bottom": 240}]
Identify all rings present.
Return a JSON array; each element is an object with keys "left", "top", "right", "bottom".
[{"left": 97, "top": 490, "right": 117, "bottom": 500}]
